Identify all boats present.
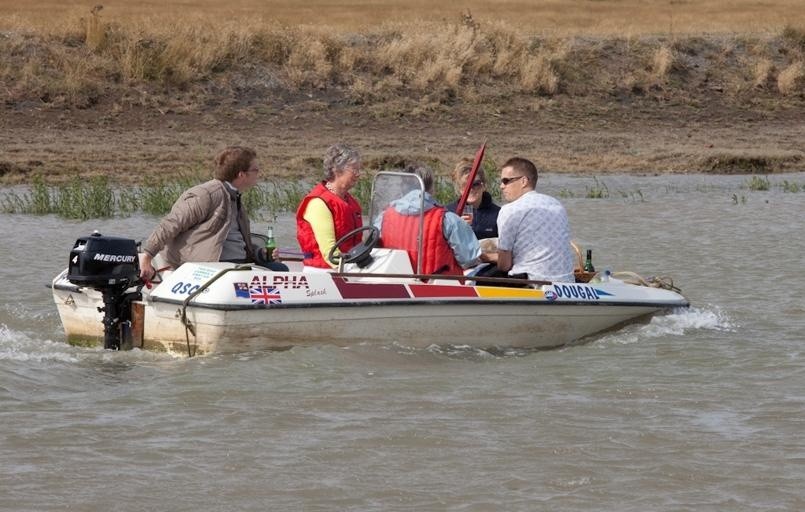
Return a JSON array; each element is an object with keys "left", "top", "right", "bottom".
[{"left": 43, "top": 169, "right": 692, "bottom": 356}]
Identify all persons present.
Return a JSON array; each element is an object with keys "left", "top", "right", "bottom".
[
  {"left": 438, "top": 153, "right": 498, "bottom": 239},
  {"left": 134, "top": 146, "right": 291, "bottom": 283},
  {"left": 465, "top": 156, "right": 577, "bottom": 288},
  {"left": 370, "top": 162, "right": 482, "bottom": 284},
  {"left": 294, "top": 144, "right": 369, "bottom": 271}
]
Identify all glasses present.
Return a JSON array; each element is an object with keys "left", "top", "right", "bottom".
[
  {"left": 240, "top": 168, "right": 260, "bottom": 177},
  {"left": 344, "top": 167, "right": 361, "bottom": 176},
  {"left": 500, "top": 176, "right": 523, "bottom": 184},
  {"left": 458, "top": 182, "right": 482, "bottom": 189}
]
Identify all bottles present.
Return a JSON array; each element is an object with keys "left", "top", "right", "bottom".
[
  {"left": 584, "top": 248, "right": 595, "bottom": 272},
  {"left": 265, "top": 225, "right": 277, "bottom": 262},
  {"left": 588, "top": 270, "right": 611, "bottom": 284}
]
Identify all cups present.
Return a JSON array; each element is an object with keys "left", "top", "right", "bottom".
[{"left": 462, "top": 204, "right": 474, "bottom": 222}]
